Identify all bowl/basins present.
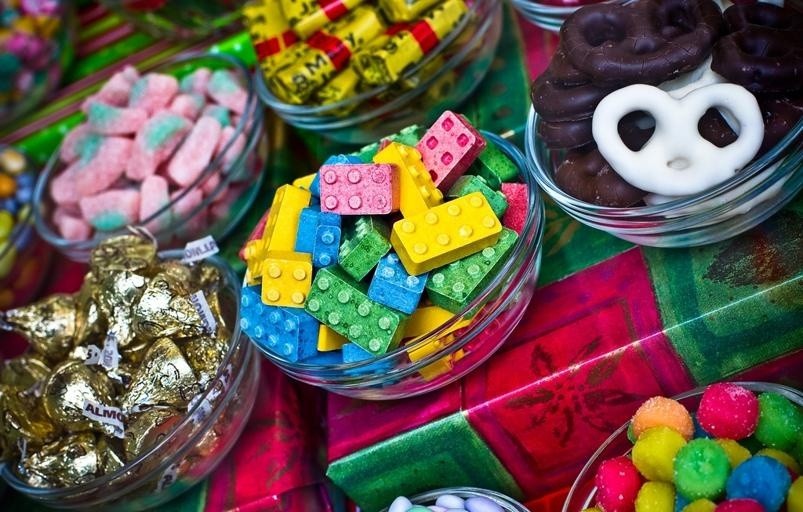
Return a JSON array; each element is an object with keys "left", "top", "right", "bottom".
[
  {"left": 101, "top": 1, "right": 241, "bottom": 43},
  {"left": 379, "top": 486, "right": 530, "bottom": 512},
  {"left": 235, "top": 131, "right": 546, "bottom": 400},
  {"left": 562, "top": 379, "right": 803, "bottom": 511},
  {"left": 253, "top": 0, "right": 504, "bottom": 147},
  {"left": 512, "top": 0, "right": 637, "bottom": 33},
  {"left": 1, "top": 248, "right": 260, "bottom": 512},
  {"left": 523, "top": 1, "right": 803, "bottom": 248},
  {"left": 1, "top": 0, "right": 74, "bottom": 124},
  {"left": 1, "top": 146, "right": 32, "bottom": 277}
]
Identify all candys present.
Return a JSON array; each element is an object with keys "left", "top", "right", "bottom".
[
  {"left": 388, "top": 490, "right": 517, "bottom": 511},
  {"left": 240, "top": 2, "right": 530, "bottom": 108},
  {"left": 0, "top": 147, "right": 35, "bottom": 252},
  {"left": 50, "top": 65, "right": 248, "bottom": 237},
  {"left": 1, "top": 225, "right": 231, "bottom": 487},
  {"left": 588, "top": 380, "right": 798, "bottom": 512},
  {"left": 237, "top": 110, "right": 528, "bottom": 382},
  {"left": 1, "top": 0, "right": 60, "bottom": 105}
]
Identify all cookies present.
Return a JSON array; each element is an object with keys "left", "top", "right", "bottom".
[{"left": 530, "top": 0, "right": 802, "bottom": 207}]
